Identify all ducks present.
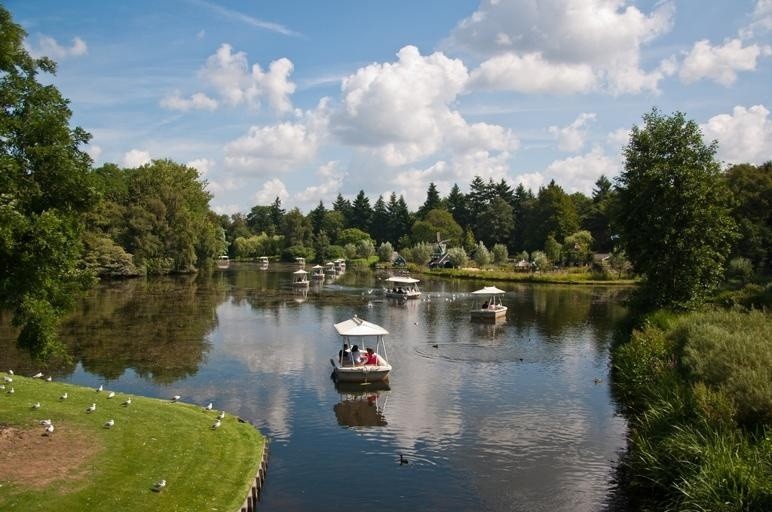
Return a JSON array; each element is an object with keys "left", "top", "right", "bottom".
[{"left": 400, "top": 454, "right": 408, "bottom": 463}]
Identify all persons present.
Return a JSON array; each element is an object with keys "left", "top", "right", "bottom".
[
  {"left": 366, "top": 348, "right": 379, "bottom": 364},
  {"left": 350, "top": 344, "right": 362, "bottom": 364},
  {"left": 339, "top": 344, "right": 348, "bottom": 363},
  {"left": 298, "top": 261, "right": 344, "bottom": 283},
  {"left": 482, "top": 301, "right": 488, "bottom": 309},
  {"left": 387, "top": 286, "right": 415, "bottom": 294},
  {"left": 490, "top": 303, "right": 494, "bottom": 309},
  {"left": 367, "top": 396, "right": 379, "bottom": 407}
]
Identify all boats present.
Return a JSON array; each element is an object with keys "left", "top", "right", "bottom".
[
  {"left": 469, "top": 286, "right": 508, "bottom": 319},
  {"left": 293, "top": 259, "right": 346, "bottom": 287},
  {"left": 384, "top": 277, "right": 422, "bottom": 299},
  {"left": 330, "top": 314, "right": 392, "bottom": 383},
  {"left": 219, "top": 256, "right": 229, "bottom": 265},
  {"left": 293, "top": 258, "right": 305, "bottom": 265},
  {"left": 256, "top": 256, "right": 268, "bottom": 263}
]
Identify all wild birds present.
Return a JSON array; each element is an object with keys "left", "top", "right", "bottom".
[
  {"left": 0, "top": 370, "right": 15, "bottom": 394},
  {"left": 32, "top": 401, "right": 40, "bottom": 410},
  {"left": 205, "top": 402, "right": 224, "bottom": 428},
  {"left": 98, "top": 383, "right": 131, "bottom": 407},
  {"left": 150, "top": 479, "right": 168, "bottom": 491},
  {"left": 33, "top": 372, "right": 53, "bottom": 382},
  {"left": 60, "top": 392, "right": 69, "bottom": 401},
  {"left": 104, "top": 419, "right": 114, "bottom": 429},
  {"left": 88, "top": 402, "right": 97, "bottom": 413},
  {"left": 170, "top": 394, "right": 181, "bottom": 402},
  {"left": 41, "top": 418, "right": 55, "bottom": 433}
]
